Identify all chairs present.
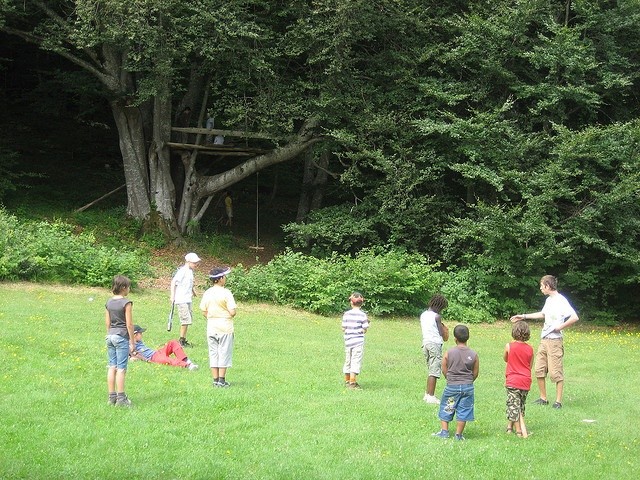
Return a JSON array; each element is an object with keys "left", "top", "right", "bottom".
[
  {"left": 533, "top": 398, "right": 549, "bottom": 406},
  {"left": 117, "top": 394, "right": 132, "bottom": 405},
  {"left": 107, "top": 394, "right": 116, "bottom": 406},
  {"left": 423, "top": 391, "right": 441, "bottom": 404},
  {"left": 553, "top": 401, "right": 561, "bottom": 409},
  {"left": 433, "top": 429, "right": 450, "bottom": 437},
  {"left": 455, "top": 434, "right": 465, "bottom": 440},
  {"left": 215, "top": 379, "right": 230, "bottom": 388},
  {"left": 188, "top": 360, "right": 199, "bottom": 370},
  {"left": 345, "top": 379, "right": 362, "bottom": 390}
]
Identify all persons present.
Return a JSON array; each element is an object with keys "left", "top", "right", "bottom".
[
  {"left": 224, "top": 193, "right": 234, "bottom": 226},
  {"left": 170, "top": 252, "right": 202, "bottom": 348},
  {"left": 204, "top": 112, "right": 215, "bottom": 144},
  {"left": 216, "top": 191, "right": 224, "bottom": 225},
  {"left": 340, "top": 293, "right": 370, "bottom": 391},
  {"left": 431, "top": 324, "right": 481, "bottom": 442},
  {"left": 503, "top": 320, "right": 535, "bottom": 438},
  {"left": 103, "top": 275, "right": 135, "bottom": 407},
  {"left": 235, "top": 186, "right": 255, "bottom": 208},
  {"left": 129, "top": 325, "right": 199, "bottom": 371},
  {"left": 510, "top": 273, "right": 580, "bottom": 409},
  {"left": 199, "top": 267, "right": 237, "bottom": 388},
  {"left": 419, "top": 294, "right": 449, "bottom": 405}
]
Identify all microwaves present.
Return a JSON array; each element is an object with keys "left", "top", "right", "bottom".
[{"left": 167, "top": 301, "right": 174, "bottom": 331}]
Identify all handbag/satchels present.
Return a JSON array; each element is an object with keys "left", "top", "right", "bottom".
[{"left": 523, "top": 314, "right": 526, "bottom": 320}]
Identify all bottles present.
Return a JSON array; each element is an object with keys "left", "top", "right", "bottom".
[
  {"left": 185, "top": 252, "right": 201, "bottom": 263},
  {"left": 207, "top": 267, "right": 231, "bottom": 279},
  {"left": 134, "top": 325, "right": 146, "bottom": 334},
  {"left": 349, "top": 294, "right": 363, "bottom": 303}
]
